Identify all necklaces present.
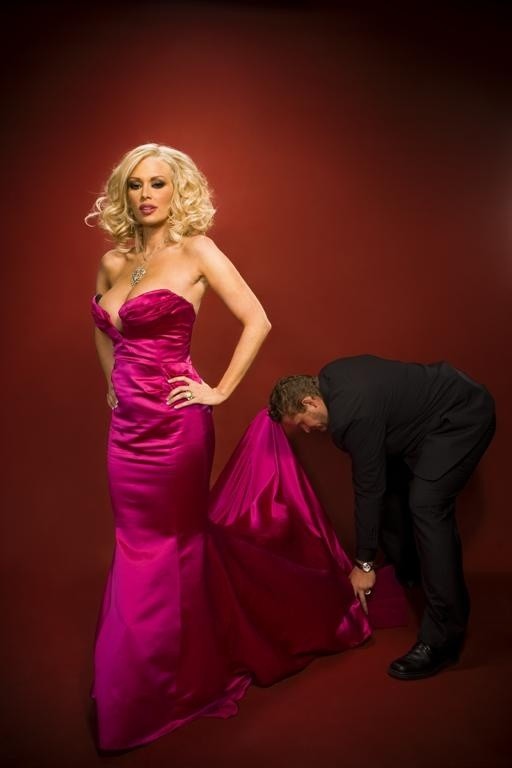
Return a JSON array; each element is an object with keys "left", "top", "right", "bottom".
[{"left": 128, "top": 237, "right": 162, "bottom": 287}]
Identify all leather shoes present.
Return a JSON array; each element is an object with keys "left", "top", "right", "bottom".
[{"left": 388, "top": 638, "right": 464, "bottom": 679}]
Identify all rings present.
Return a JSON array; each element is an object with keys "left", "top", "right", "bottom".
[{"left": 185, "top": 390, "right": 193, "bottom": 401}]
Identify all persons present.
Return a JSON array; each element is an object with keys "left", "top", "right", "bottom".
[
  {"left": 90, "top": 141, "right": 272, "bottom": 756},
  {"left": 266, "top": 353, "right": 497, "bottom": 682}
]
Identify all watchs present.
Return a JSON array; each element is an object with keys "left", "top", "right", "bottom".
[{"left": 351, "top": 559, "right": 374, "bottom": 573}]
